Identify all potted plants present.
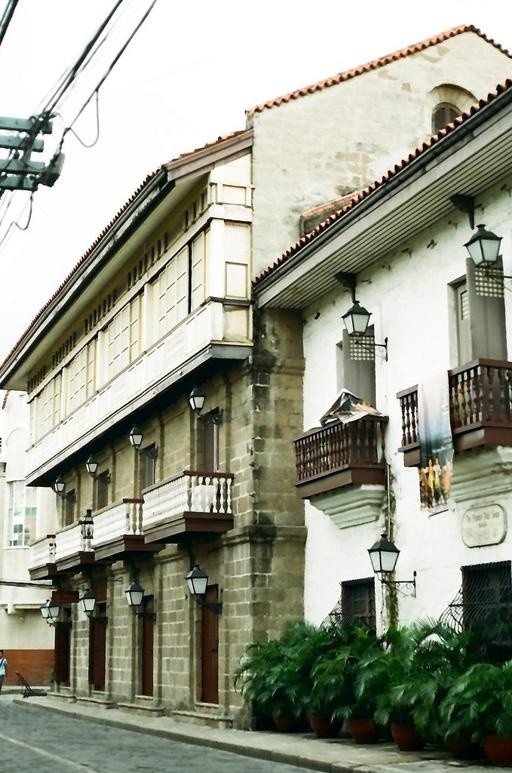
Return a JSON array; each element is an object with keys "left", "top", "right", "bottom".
[{"left": 233, "top": 619, "right": 512, "bottom": 764}]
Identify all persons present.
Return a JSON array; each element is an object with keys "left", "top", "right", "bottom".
[
  {"left": 0, "top": 648, "right": 8, "bottom": 698},
  {"left": 419, "top": 453, "right": 452, "bottom": 510}
]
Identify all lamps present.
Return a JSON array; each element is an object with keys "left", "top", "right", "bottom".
[
  {"left": 86, "top": 456, "right": 110, "bottom": 481},
  {"left": 185, "top": 564, "right": 223, "bottom": 612},
  {"left": 124, "top": 578, "right": 155, "bottom": 620},
  {"left": 40, "top": 600, "right": 72, "bottom": 627},
  {"left": 80, "top": 588, "right": 107, "bottom": 623},
  {"left": 342, "top": 300, "right": 389, "bottom": 349},
  {"left": 370, "top": 534, "right": 416, "bottom": 588},
  {"left": 189, "top": 389, "right": 222, "bottom": 423},
  {"left": 130, "top": 427, "right": 159, "bottom": 458},
  {"left": 52, "top": 477, "right": 74, "bottom": 500},
  {"left": 466, "top": 226, "right": 511, "bottom": 282}
]
